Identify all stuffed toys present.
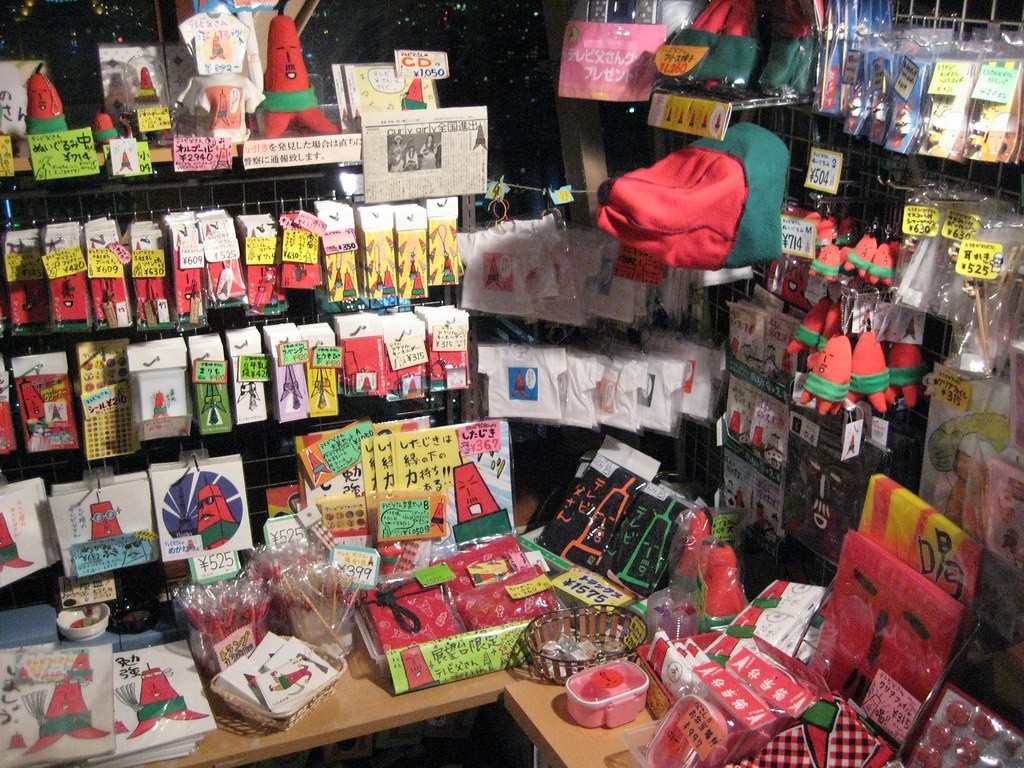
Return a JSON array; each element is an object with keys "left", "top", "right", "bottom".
[
  {"left": 260, "top": 16, "right": 340, "bottom": 139},
  {"left": 673, "top": 510, "right": 748, "bottom": 633},
  {"left": 788, "top": 210, "right": 930, "bottom": 413},
  {"left": 92, "top": 113, "right": 118, "bottom": 146},
  {"left": 24, "top": 72, "right": 70, "bottom": 135}
]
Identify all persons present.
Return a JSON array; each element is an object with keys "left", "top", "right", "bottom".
[{"left": 388, "top": 134, "right": 439, "bottom": 170}]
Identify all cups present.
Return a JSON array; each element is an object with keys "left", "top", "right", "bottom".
[
  {"left": 287, "top": 605, "right": 355, "bottom": 658},
  {"left": 187, "top": 608, "right": 268, "bottom": 670}
]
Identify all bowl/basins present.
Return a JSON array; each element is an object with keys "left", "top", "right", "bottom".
[
  {"left": 566, "top": 660, "right": 649, "bottom": 728},
  {"left": 645, "top": 694, "right": 728, "bottom": 768},
  {"left": 57, "top": 603, "right": 110, "bottom": 641},
  {"left": 110, "top": 595, "right": 159, "bottom": 634}
]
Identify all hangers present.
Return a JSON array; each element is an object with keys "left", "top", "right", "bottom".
[{"left": 489, "top": 196, "right": 697, "bottom": 358}]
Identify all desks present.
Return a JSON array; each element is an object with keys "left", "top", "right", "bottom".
[
  {"left": 504, "top": 668, "right": 658, "bottom": 768},
  {"left": 128, "top": 612, "right": 576, "bottom": 768}
]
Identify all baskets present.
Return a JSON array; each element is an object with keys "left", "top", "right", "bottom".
[{"left": 524, "top": 603, "right": 649, "bottom": 687}]
